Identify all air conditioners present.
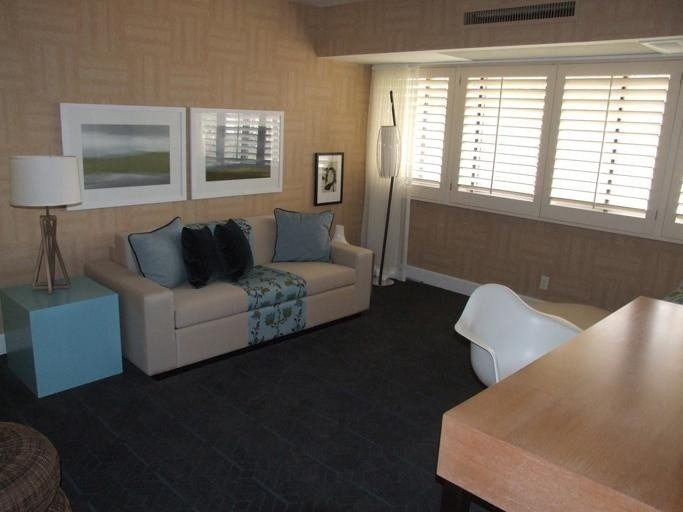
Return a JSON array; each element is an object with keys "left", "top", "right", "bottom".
[{"left": 462, "top": 0, "right": 578, "bottom": 29}]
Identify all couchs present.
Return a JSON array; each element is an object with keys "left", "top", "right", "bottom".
[{"left": 84, "top": 212, "right": 374, "bottom": 377}]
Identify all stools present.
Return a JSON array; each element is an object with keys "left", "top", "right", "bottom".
[{"left": 0, "top": 420, "right": 61, "bottom": 512}]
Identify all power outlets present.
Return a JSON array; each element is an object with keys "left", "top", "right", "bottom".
[{"left": 539, "top": 275, "right": 550, "bottom": 291}]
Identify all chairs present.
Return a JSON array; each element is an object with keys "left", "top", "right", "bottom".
[{"left": 453, "top": 280, "right": 584, "bottom": 387}]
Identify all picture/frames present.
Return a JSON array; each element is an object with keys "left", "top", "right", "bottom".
[
  {"left": 59, "top": 102, "right": 187, "bottom": 210},
  {"left": 313, "top": 151, "right": 343, "bottom": 206},
  {"left": 190, "top": 106, "right": 288, "bottom": 200}
]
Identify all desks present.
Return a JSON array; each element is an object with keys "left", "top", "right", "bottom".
[{"left": 435, "top": 295, "right": 683, "bottom": 512}]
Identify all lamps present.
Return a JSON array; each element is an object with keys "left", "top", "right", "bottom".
[
  {"left": 371, "top": 87, "right": 407, "bottom": 288},
  {"left": 9, "top": 156, "right": 84, "bottom": 293}
]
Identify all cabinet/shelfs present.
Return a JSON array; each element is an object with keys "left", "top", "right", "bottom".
[{"left": 0, "top": 277, "right": 124, "bottom": 400}]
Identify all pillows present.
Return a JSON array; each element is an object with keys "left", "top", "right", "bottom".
[
  {"left": 271, "top": 206, "right": 334, "bottom": 266},
  {"left": 215, "top": 217, "right": 255, "bottom": 282},
  {"left": 179, "top": 221, "right": 215, "bottom": 290},
  {"left": 127, "top": 214, "right": 194, "bottom": 286}
]
